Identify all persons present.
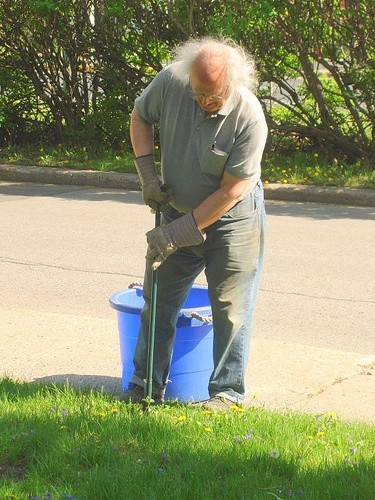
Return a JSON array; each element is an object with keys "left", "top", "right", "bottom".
[{"left": 109, "top": 37, "right": 268, "bottom": 414}]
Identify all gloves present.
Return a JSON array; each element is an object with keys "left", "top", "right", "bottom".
[
  {"left": 145, "top": 211, "right": 207, "bottom": 270},
  {"left": 134, "top": 154, "right": 173, "bottom": 212}
]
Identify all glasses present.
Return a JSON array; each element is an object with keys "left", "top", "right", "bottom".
[{"left": 188, "top": 75, "right": 231, "bottom": 99}]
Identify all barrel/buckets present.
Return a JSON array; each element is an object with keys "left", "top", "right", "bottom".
[{"left": 109, "top": 285, "right": 214, "bottom": 403}]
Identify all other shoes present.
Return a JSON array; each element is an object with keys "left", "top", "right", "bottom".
[
  {"left": 120, "top": 383, "right": 164, "bottom": 404},
  {"left": 201, "top": 397, "right": 236, "bottom": 411}
]
